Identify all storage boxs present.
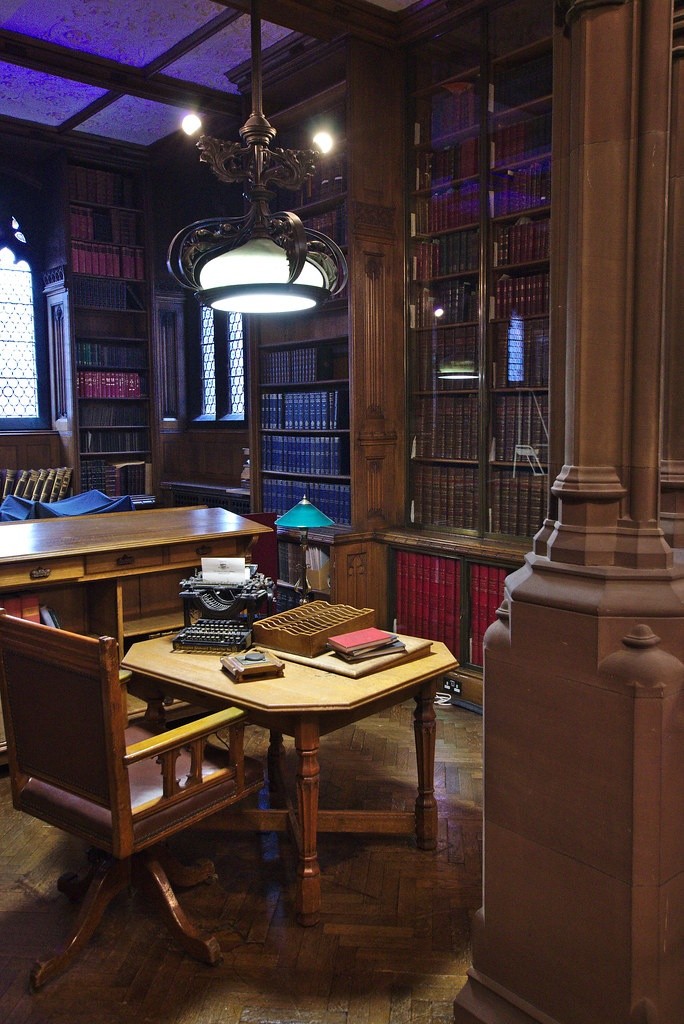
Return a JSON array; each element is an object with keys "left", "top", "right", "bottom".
[{"left": 307, "top": 559, "right": 330, "bottom": 591}]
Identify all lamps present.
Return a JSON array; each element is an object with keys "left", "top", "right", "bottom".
[{"left": 166, "top": 0, "right": 349, "bottom": 315}]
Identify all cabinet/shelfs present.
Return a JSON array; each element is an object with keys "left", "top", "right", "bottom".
[
  {"left": 375, "top": 31, "right": 550, "bottom": 717},
  {"left": 40, "top": 166, "right": 156, "bottom": 499},
  {"left": 242, "top": 122, "right": 352, "bottom": 607},
  {"left": 0, "top": 507, "right": 277, "bottom": 663}
]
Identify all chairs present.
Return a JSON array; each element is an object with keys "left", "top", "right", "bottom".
[{"left": 0, "top": 608, "right": 265, "bottom": 996}]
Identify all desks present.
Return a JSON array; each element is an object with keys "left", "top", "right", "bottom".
[
  {"left": 120, "top": 629, "right": 460, "bottom": 927},
  {"left": 158, "top": 471, "right": 251, "bottom": 515}
]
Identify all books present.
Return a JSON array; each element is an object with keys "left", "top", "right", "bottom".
[
  {"left": 260, "top": 347, "right": 333, "bottom": 384},
  {"left": 2, "top": 165, "right": 153, "bottom": 503},
  {"left": 294, "top": 151, "right": 345, "bottom": 209},
  {"left": 411, "top": 53, "right": 553, "bottom": 538},
  {"left": 302, "top": 211, "right": 339, "bottom": 245},
  {"left": 0, "top": 590, "right": 62, "bottom": 629},
  {"left": 260, "top": 389, "right": 349, "bottom": 429},
  {"left": 249, "top": 540, "right": 508, "bottom": 680},
  {"left": 261, "top": 434, "right": 351, "bottom": 526}
]
